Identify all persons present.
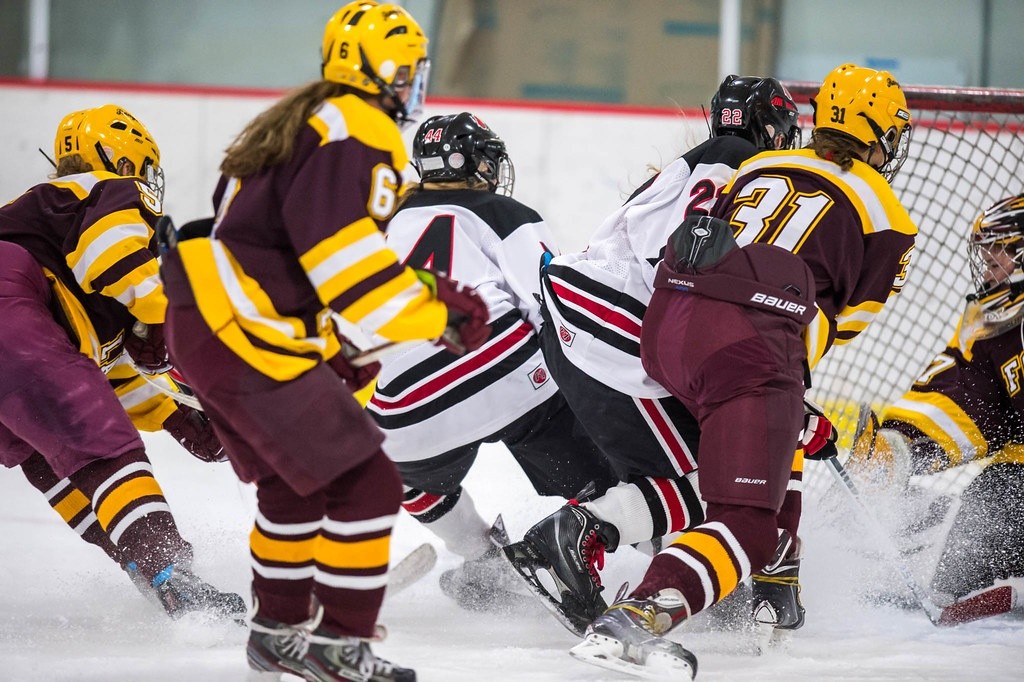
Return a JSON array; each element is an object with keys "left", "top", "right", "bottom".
[
  {"left": 161, "top": 0, "right": 492, "bottom": 682},
  {"left": 498, "top": 75, "right": 800, "bottom": 645},
  {"left": 876, "top": 194, "right": 1023, "bottom": 629},
  {"left": 358, "top": 111, "right": 751, "bottom": 637},
  {"left": 0, "top": 104, "right": 248, "bottom": 643},
  {"left": 568, "top": 62, "right": 916, "bottom": 682}
]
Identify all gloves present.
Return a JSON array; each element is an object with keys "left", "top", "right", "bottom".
[
  {"left": 433, "top": 274, "right": 492, "bottom": 355},
  {"left": 796, "top": 401, "right": 838, "bottom": 461},
  {"left": 328, "top": 318, "right": 380, "bottom": 391},
  {"left": 123, "top": 321, "right": 175, "bottom": 376},
  {"left": 163, "top": 403, "right": 230, "bottom": 464}
]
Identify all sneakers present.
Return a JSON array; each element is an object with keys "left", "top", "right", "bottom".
[
  {"left": 994, "top": 575, "right": 1024, "bottom": 610},
  {"left": 499, "top": 484, "right": 620, "bottom": 638},
  {"left": 438, "top": 545, "right": 554, "bottom": 624},
  {"left": 245, "top": 617, "right": 415, "bottom": 682},
  {"left": 706, "top": 526, "right": 803, "bottom": 649},
  {"left": 123, "top": 549, "right": 254, "bottom": 649},
  {"left": 570, "top": 583, "right": 698, "bottom": 682}
]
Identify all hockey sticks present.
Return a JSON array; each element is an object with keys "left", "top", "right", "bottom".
[
  {"left": 826, "top": 456, "right": 1018, "bottom": 631},
  {"left": 133, "top": 320, "right": 439, "bottom": 600}
]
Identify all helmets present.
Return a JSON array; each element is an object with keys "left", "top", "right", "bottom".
[
  {"left": 966, "top": 196, "right": 1024, "bottom": 296},
  {"left": 710, "top": 74, "right": 802, "bottom": 151},
  {"left": 319, "top": 2, "right": 430, "bottom": 134},
  {"left": 813, "top": 65, "right": 914, "bottom": 184},
  {"left": 55, "top": 104, "right": 164, "bottom": 206},
  {"left": 411, "top": 112, "right": 516, "bottom": 197}
]
